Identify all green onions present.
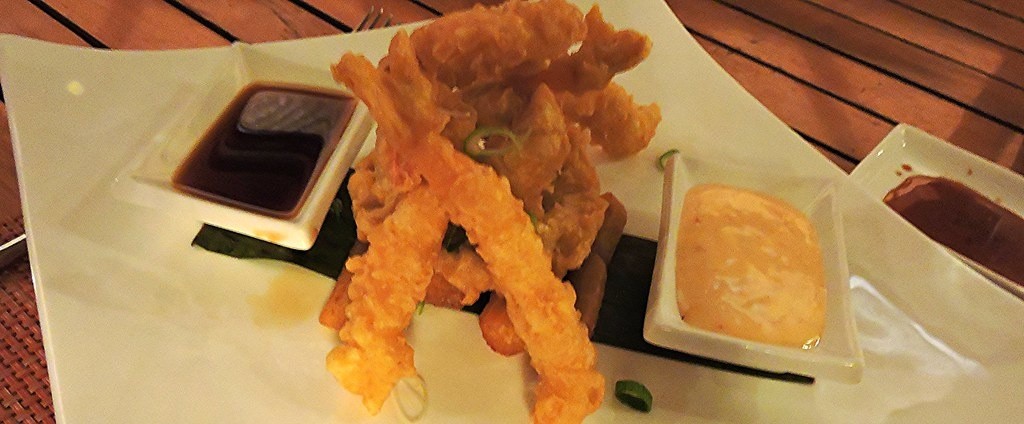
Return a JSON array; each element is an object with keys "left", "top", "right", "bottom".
[{"left": 393, "top": 126, "right": 681, "bottom": 422}]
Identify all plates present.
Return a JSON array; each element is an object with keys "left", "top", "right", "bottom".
[
  {"left": 642, "top": 153, "right": 865, "bottom": 383},
  {"left": 0, "top": 0, "right": 1024, "bottom": 424},
  {"left": 851, "top": 124, "right": 1024, "bottom": 294},
  {"left": 112, "top": 41, "right": 372, "bottom": 251}
]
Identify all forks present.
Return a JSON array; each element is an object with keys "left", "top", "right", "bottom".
[{"left": 0, "top": 8, "right": 402, "bottom": 270}]
